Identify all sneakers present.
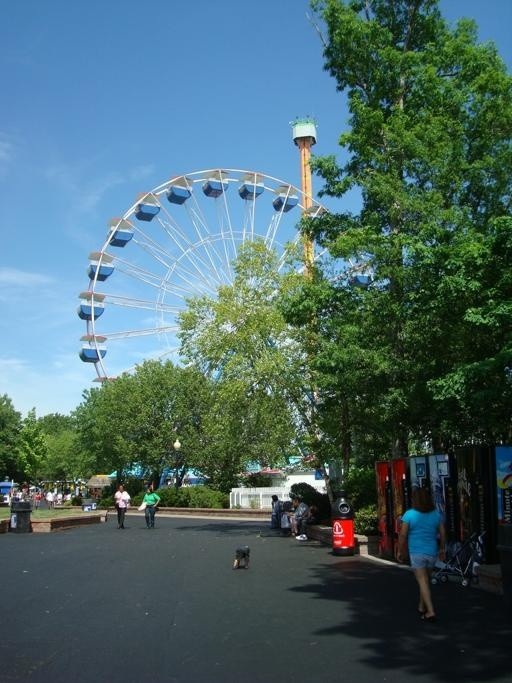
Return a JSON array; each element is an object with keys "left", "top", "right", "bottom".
[{"left": 296, "top": 534, "right": 307, "bottom": 540}]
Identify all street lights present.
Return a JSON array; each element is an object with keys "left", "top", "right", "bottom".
[{"left": 173, "top": 439, "right": 181, "bottom": 494}]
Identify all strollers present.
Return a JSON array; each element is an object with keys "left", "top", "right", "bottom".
[{"left": 430, "top": 529, "right": 487, "bottom": 587}]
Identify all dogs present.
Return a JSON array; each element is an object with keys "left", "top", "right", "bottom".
[{"left": 232, "top": 546, "right": 252, "bottom": 569}]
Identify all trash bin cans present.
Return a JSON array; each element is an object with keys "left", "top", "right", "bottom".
[
  {"left": 331, "top": 496, "right": 355, "bottom": 556},
  {"left": 9, "top": 503, "right": 32, "bottom": 533}
]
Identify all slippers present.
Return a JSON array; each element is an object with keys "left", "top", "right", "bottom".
[{"left": 421, "top": 613, "right": 435, "bottom": 621}]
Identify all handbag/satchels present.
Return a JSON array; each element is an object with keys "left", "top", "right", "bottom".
[{"left": 127, "top": 502, "right": 131, "bottom": 509}]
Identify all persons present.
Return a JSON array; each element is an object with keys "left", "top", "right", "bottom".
[
  {"left": 9, "top": 488, "right": 69, "bottom": 510},
  {"left": 269, "top": 494, "right": 321, "bottom": 541},
  {"left": 395, "top": 486, "right": 448, "bottom": 624},
  {"left": 142, "top": 486, "right": 161, "bottom": 529},
  {"left": 113, "top": 484, "right": 132, "bottom": 529}
]
